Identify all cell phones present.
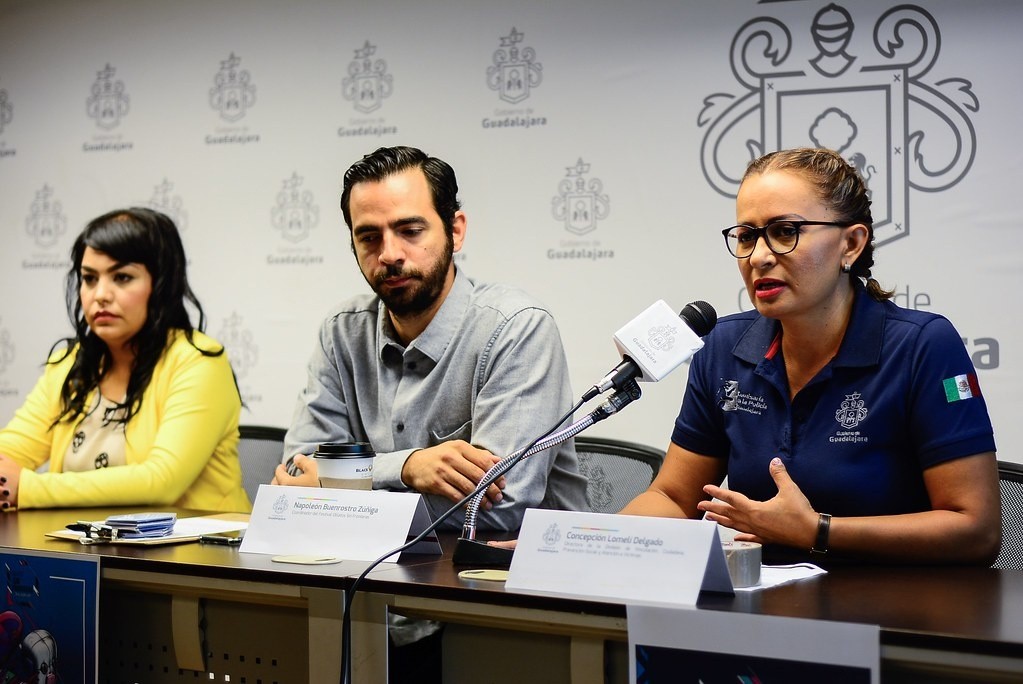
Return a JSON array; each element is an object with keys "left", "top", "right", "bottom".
[{"left": 198, "top": 528, "right": 247, "bottom": 546}]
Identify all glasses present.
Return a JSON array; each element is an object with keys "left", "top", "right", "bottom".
[{"left": 722, "top": 220, "right": 854, "bottom": 260}]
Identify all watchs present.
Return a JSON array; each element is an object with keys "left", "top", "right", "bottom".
[{"left": 810, "top": 512, "right": 831, "bottom": 562}]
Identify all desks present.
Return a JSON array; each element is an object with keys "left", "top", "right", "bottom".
[{"left": 0, "top": 487, "right": 1023, "bottom": 683}]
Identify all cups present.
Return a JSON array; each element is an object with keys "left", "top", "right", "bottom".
[
  {"left": 720, "top": 540, "right": 762, "bottom": 589},
  {"left": 314, "top": 442, "right": 376, "bottom": 491}
]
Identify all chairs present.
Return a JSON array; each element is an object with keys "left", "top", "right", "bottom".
[
  {"left": 570, "top": 437, "right": 668, "bottom": 512},
  {"left": 989, "top": 459, "right": 1023, "bottom": 572},
  {"left": 238, "top": 425, "right": 289, "bottom": 506}
]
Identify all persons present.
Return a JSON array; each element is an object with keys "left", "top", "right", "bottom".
[
  {"left": 270, "top": 146, "right": 590, "bottom": 645},
  {"left": 486, "top": 148, "right": 1005, "bottom": 575},
  {"left": 0, "top": 207, "right": 253, "bottom": 514}
]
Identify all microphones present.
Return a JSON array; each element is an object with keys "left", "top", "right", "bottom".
[{"left": 582, "top": 299, "right": 718, "bottom": 403}]
